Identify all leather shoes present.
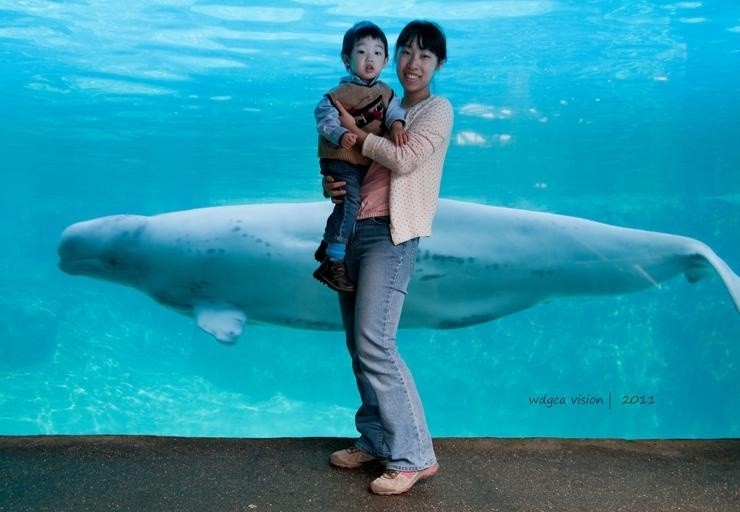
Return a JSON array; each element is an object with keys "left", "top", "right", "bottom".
[{"left": 313, "top": 240, "right": 355, "bottom": 292}]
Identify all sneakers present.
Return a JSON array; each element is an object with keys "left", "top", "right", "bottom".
[
  {"left": 330, "top": 445, "right": 375, "bottom": 468},
  {"left": 369, "top": 460, "right": 441, "bottom": 495}
]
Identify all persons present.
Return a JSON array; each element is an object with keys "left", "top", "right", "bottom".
[
  {"left": 312, "top": 21, "right": 410, "bottom": 294},
  {"left": 322, "top": 20, "right": 453, "bottom": 494}
]
